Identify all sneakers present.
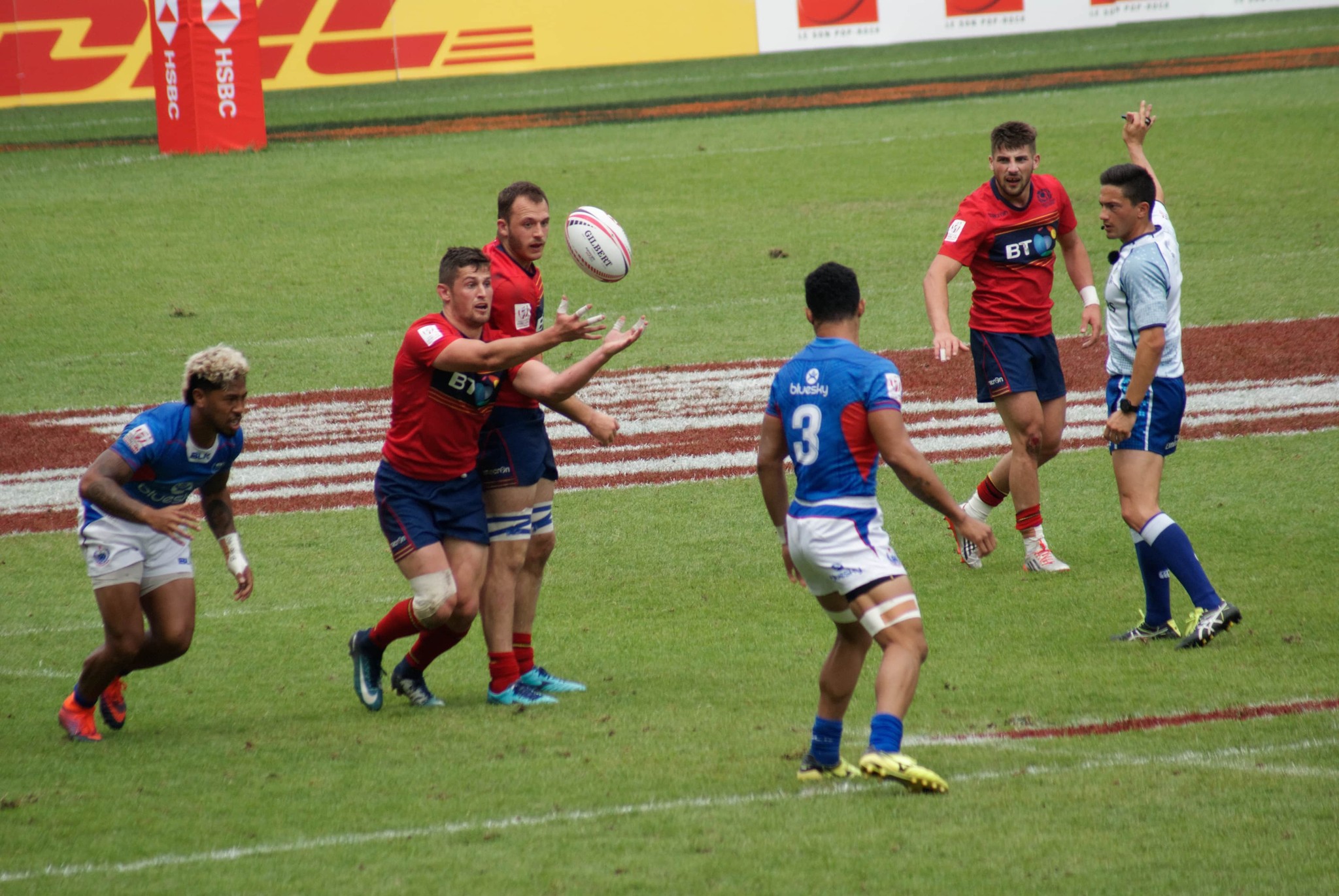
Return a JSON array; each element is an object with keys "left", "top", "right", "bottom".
[
  {"left": 57, "top": 692, "right": 102, "bottom": 743},
  {"left": 100, "top": 675, "right": 129, "bottom": 730},
  {"left": 485, "top": 682, "right": 561, "bottom": 706},
  {"left": 859, "top": 750, "right": 949, "bottom": 793},
  {"left": 389, "top": 659, "right": 447, "bottom": 708},
  {"left": 944, "top": 502, "right": 993, "bottom": 567},
  {"left": 348, "top": 627, "right": 385, "bottom": 711},
  {"left": 1110, "top": 607, "right": 1186, "bottom": 642},
  {"left": 1023, "top": 539, "right": 1076, "bottom": 574},
  {"left": 522, "top": 664, "right": 589, "bottom": 693},
  {"left": 796, "top": 753, "right": 865, "bottom": 780},
  {"left": 1176, "top": 597, "right": 1243, "bottom": 650}
]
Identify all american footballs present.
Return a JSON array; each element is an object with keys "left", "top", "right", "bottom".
[{"left": 564, "top": 203, "right": 639, "bottom": 287}]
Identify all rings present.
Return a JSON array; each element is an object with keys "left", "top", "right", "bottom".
[{"left": 1145, "top": 117, "right": 1151, "bottom": 125}]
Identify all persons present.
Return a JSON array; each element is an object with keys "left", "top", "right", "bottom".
[
  {"left": 348, "top": 244, "right": 646, "bottom": 712},
  {"left": 756, "top": 263, "right": 998, "bottom": 796},
  {"left": 478, "top": 180, "right": 618, "bottom": 707},
  {"left": 1099, "top": 101, "right": 1243, "bottom": 649},
  {"left": 57, "top": 342, "right": 255, "bottom": 739},
  {"left": 923, "top": 121, "right": 1103, "bottom": 573}
]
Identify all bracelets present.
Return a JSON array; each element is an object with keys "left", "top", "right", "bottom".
[{"left": 1078, "top": 285, "right": 1101, "bottom": 306}]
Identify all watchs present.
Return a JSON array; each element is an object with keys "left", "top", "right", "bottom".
[{"left": 1121, "top": 398, "right": 1141, "bottom": 413}]
